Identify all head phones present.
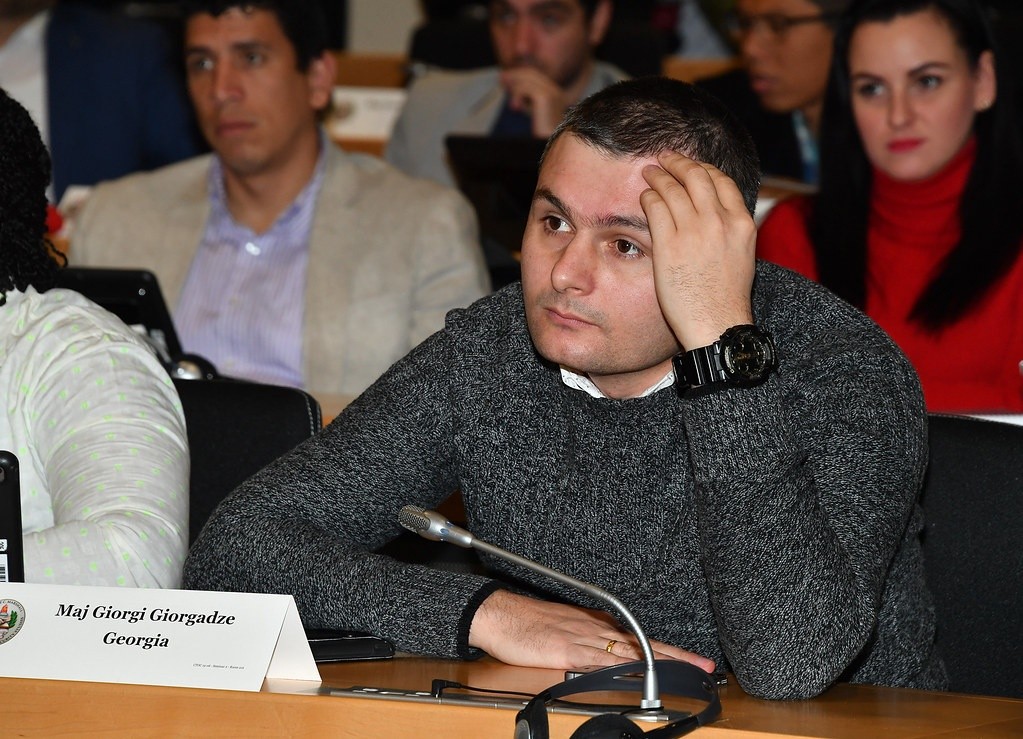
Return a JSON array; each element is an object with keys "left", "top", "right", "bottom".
[{"left": 512, "top": 657, "right": 721, "bottom": 739}]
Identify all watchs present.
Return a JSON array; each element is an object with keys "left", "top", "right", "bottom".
[{"left": 671, "top": 323, "right": 780, "bottom": 400}]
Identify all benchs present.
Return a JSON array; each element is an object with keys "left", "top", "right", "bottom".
[{"left": 0, "top": 636, "right": 1023, "bottom": 739}]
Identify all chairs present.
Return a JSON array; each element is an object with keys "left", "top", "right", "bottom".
[
  {"left": 916, "top": 411, "right": 1023, "bottom": 700},
  {"left": 168, "top": 378, "right": 321, "bottom": 565}
]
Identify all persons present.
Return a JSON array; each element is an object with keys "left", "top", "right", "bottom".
[
  {"left": 51, "top": 2, "right": 494, "bottom": 426},
  {"left": 754, "top": 0, "right": 1023, "bottom": 432},
  {"left": 0, "top": 81, "right": 191, "bottom": 589},
  {"left": 0, "top": 0, "right": 193, "bottom": 206},
  {"left": 379, "top": 0, "right": 844, "bottom": 294},
  {"left": 179, "top": 76, "right": 952, "bottom": 703}
]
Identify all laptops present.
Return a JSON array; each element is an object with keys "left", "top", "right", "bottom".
[
  {"left": 0, "top": 449, "right": 26, "bottom": 583},
  {"left": 444, "top": 131, "right": 551, "bottom": 254},
  {"left": 54, "top": 266, "right": 184, "bottom": 379}
]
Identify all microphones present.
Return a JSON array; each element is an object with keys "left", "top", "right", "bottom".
[{"left": 395, "top": 506, "right": 693, "bottom": 721}]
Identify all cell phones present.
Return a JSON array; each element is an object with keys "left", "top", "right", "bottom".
[{"left": 564, "top": 663, "right": 728, "bottom": 693}]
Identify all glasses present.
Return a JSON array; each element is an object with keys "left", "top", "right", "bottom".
[{"left": 723, "top": 9, "right": 823, "bottom": 40}]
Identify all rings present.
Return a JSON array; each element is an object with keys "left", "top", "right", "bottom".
[{"left": 606, "top": 639, "right": 617, "bottom": 654}]
